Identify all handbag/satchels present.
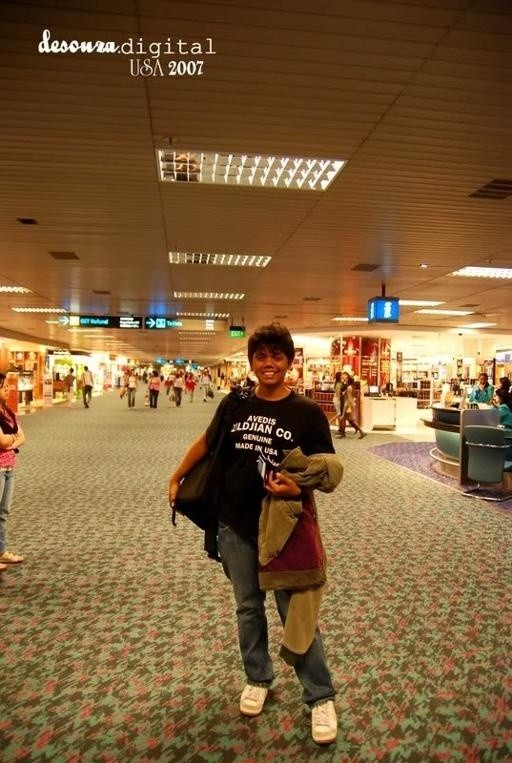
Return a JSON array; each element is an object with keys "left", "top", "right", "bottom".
[{"left": 177, "top": 438, "right": 226, "bottom": 553}]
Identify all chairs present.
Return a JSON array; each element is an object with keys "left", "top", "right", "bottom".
[{"left": 461, "top": 425, "right": 512, "bottom": 501}]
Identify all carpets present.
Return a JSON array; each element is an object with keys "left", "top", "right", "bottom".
[{"left": 363, "top": 440, "right": 512, "bottom": 513}]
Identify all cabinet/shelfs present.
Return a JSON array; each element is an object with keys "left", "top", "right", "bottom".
[
  {"left": 11, "top": 351, "right": 44, "bottom": 401},
  {"left": 307, "top": 358, "right": 443, "bottom": 430},
  {"left": 331, "top": 336, "right": 392, "bottom": 395}
]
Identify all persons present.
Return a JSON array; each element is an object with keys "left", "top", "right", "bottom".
[
  {"left": 116, "top": 361, "right": 216, "bottom": 408},
  {"left": 486, "top": 388, "right": 511, "bottom": 495},
  {"left": 63, "top": 368, "right": 76, "bottom": 408},
  {"left": 469, "top": 373, "right": 494, "bottom": 403},
  {"left": 498, "top": 377, "right": 511, "bottom": 387},
  {"left": 328, "top": 371, "right": 368, "bottom": 439},
  {"left": 79, "top": 366, "right": 95, "bottom": 408},
  {"left": 0, "top": 373, "right": 25, "bottom": 572},
  {"left": 163, "top": 320, "right": 344, "bottom": 743}
]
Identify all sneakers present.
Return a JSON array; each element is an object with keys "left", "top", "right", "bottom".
[
  {"left": 240, "top": 682, "right": 268, "bottom": 716},
  {"left": 0, "top": 551, "right": 24, "bottom": 563},
  {"left": 311, "top": 699, "right": 338, "bottom": 744}
]
